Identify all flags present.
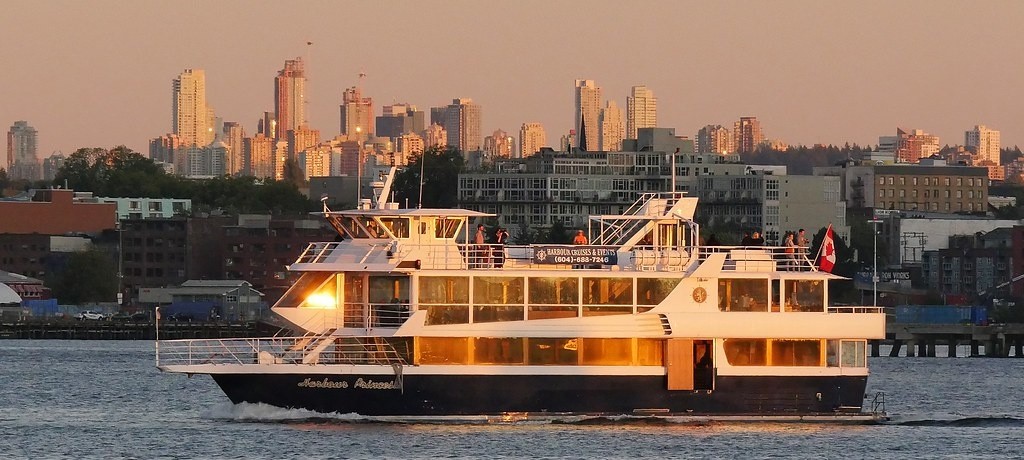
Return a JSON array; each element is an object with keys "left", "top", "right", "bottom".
[{"left": 819, "top": 224, "right": 836, "bottom": 273}]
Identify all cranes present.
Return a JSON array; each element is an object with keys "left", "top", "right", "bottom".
[{"left": 354, "top": 72, "right": 367, "bottom": 142}]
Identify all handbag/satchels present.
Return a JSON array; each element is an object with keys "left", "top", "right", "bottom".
[{"left": 785, "top": 242, "right": 794, "bottom": 253}]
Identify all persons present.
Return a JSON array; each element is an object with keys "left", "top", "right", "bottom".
[
  {"left": 706, "top": 234, "right": 725, "bottom": 270},
  {"left": 474, "top": 224, "right": 485, "bottom": 268},
  {"left": 785, "top": 233, "right": 800, "bottom": 273},
  {"left": 742, "top": 230, "right": 765, "bottom": 250},
  {"left": 573, "top": 229, "right": 588, "bottom": 271},
  {"left": 797, "top": 229, "right": 808, "bottom": 272},
  {"left": 366, "top": 224, "right": 377, "bottom": 238},
  {"left": 492, "top": 228, "right": 509, "bottom": 268}
]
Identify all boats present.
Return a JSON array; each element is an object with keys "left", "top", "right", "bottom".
[{"left": 156, "top": 145, "right": 895, "bottom": 424}]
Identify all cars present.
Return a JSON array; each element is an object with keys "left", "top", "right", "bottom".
[
  {"left": 104, "top": 310, "right": 156, "bottom": 325},
  {"left": 74, "top": 309, "right": 105, "bottom": 322},
  {"left": 166, "top": 311, "right": 193, "bottom": 324}
]
